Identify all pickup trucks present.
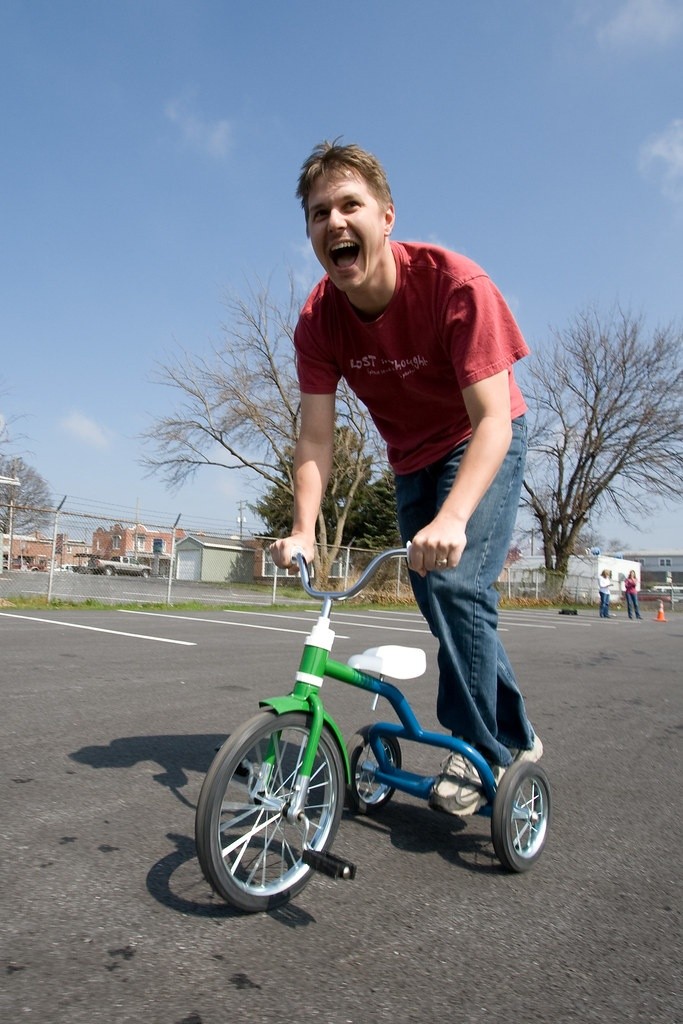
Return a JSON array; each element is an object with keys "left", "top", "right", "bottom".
[{"left": 88, "top": 555, "right": 153, "bottom": 579}]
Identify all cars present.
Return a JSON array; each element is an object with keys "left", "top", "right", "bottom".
[{"left": 3, "top": 560, "right": 45, "bottom": 571}]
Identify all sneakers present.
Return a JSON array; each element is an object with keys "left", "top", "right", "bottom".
[{"left": 427, "top": 734, "right": 543, "bottom": 816}]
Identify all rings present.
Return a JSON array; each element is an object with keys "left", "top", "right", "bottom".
[{"left": 438, "top": 559, "right": 448, "bottom": 566}]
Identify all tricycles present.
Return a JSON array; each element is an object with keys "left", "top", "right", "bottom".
[{"left": 193, "top": 541, "right": 551, "bottom": 913}]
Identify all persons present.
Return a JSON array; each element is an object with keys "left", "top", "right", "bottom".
[
  {"left": 597, "top": 569, "right": 614, "bottom": 617},
  {"left": 267, "top": 137, "right": 545, "bottom": 817},
  {"left": 625, "top": 570, "right": 643, "bottom": 620}
]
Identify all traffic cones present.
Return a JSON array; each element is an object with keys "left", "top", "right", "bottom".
[{"left": 652, "top": 600, "right": 669, "bottom": 622}]
